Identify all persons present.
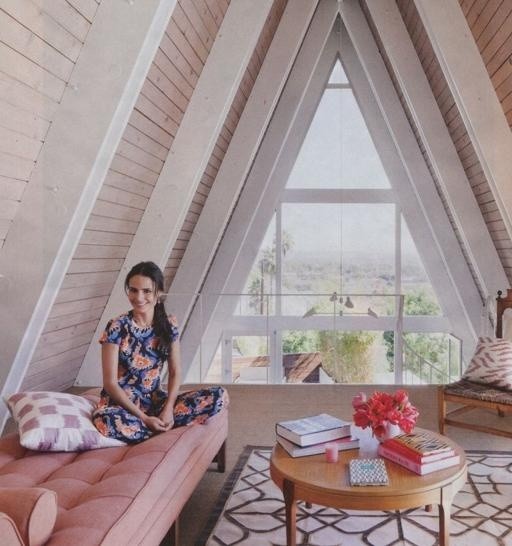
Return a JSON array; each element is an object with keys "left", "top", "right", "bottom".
[{"left": 92, "top": 261, "right": 232, "bottom": 444}]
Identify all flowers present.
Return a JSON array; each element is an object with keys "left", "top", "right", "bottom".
[{"left": 352, "top": 389, "right": 420, "bottom": 437}]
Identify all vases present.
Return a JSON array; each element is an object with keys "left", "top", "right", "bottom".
[{"left": 372, "top": 421, "right": 401, "bottom": 444}]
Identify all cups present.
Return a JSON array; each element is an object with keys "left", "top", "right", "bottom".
[{"left": 326, "top": 442, "right": 338, "bottom": 463}]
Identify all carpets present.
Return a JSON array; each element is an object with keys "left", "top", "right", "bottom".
[{"left": 193, "top": 446, "right": 511, "bottom": 546}]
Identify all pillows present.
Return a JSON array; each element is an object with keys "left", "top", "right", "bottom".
[
  {"left": 0, "top": 487, "right": 58, "bottom": 546},
  {"left": 1, "top": 391, "right": 128, "bottom": 451},
  {"left": 462, "top": 335, "right": 511, "bottom": 392}
]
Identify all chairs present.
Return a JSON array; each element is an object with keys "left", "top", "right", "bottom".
[{"left": 438, "top": 289, "right": 511, "bottom": 440}]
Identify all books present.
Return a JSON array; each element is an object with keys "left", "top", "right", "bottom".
[
  {"left": 274, "top": 412, "right": 352, "bottom": 449},
  {"left": 276, "top": 434, "right": 359, "bottom": 458},
  {"left": 348, "top": 459, "right": 389, "bottom": 486},
  {"left": 392, "top": 431, "right": 452, "bottom": 456},
  {"left": 382, "top": 437, "right": 456, "bottom": 464},
  {"left": 377, "top": 446, "right": 461, "bottom": 476}
]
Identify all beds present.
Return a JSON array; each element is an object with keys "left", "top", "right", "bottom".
[{"left": 0, "top": 384, "right": 229, "bottom": 546}]
